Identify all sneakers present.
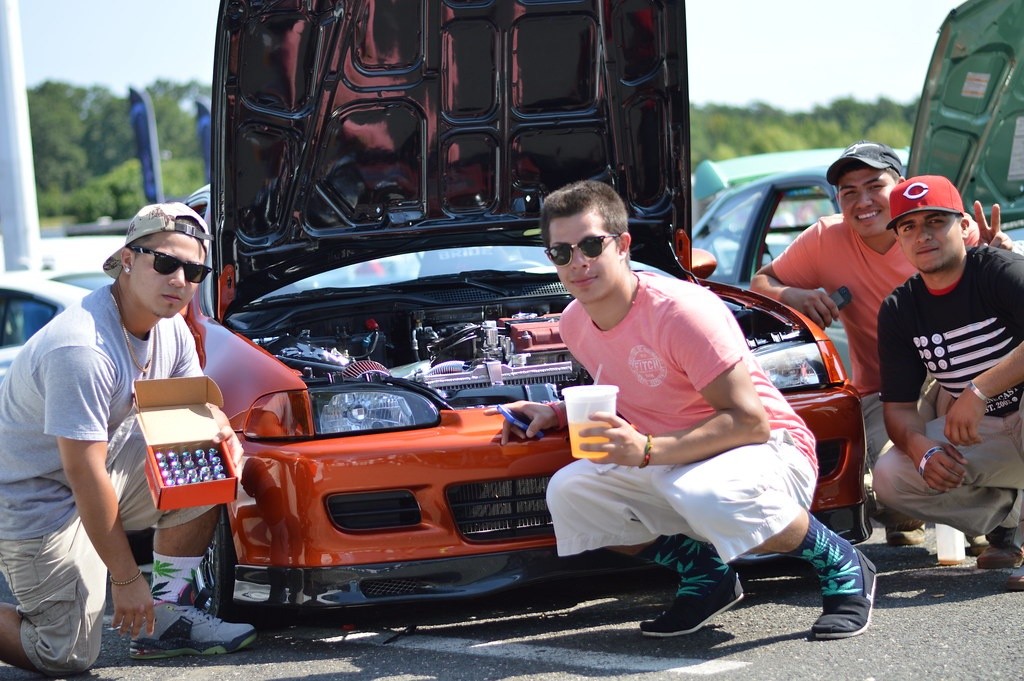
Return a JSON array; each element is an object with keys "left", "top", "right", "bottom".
[{"left": 129, "top": 600, "right": 258, "bottom": 660}]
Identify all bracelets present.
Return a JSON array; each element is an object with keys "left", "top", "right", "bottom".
[
  {"left": 638, "top": 433, "right": 653, "bottom": 469},
  {"left": 110, "top": 569, "right": 142, "bottom": 586},
  {"left": 966, "top": 379, "right": 995, "bottom": 406},
  {"left": 545, "top": 402, "right": 566, "bottom": 431},
  {"left": 918, "top": 446, "right": 944, "bottom": 479}
]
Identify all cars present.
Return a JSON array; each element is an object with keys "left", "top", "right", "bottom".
[
  {"left": 691, "top": 0, "right": 1024, "bottom": 383},
  {"left": 0, "top": 237, "right": 127, "bottom": 376},
  {"left": 175, "top": 0, "right": 872, "bottom": 635}
]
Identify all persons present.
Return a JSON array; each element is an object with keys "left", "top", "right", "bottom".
[
  {"left": 481, "top": 178, "right": 884, "bottom": 641},
  {"left": 748, "top": 140, "right": 1015, "bottom": 546},
  {"left": 871, "top": 173, "right": 1024, "bottom": 571},
  {"left": 0, "top": 200, "right": 261, "bottom": 679}
]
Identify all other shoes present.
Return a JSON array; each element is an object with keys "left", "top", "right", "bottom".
[
  {"left": 966, "top": 534, "right": 991, "bottom": 554},
  {"left": 885, "top": 522, "right": 925, "bottom": 544},
  {"left": 639, "top": 565, "right": 744, "bottom": 637},
  {"left": 977, "top": 526, "right": 1024, "bottom": 568},
  {"left": 1006, "top": 564, "right": 1024, "bottom": 590},
  {"left": 811, "top": 544, "right": 877, "bottom": 638}
]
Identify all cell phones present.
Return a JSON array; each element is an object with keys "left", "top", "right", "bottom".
[
  {"left": 497, "top": 404, "right": 543, "bottom": 438},
  {"left": 828, "top": 286, "right": 852, "bottom": 309}
]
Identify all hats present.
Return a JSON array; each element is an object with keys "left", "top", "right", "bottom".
[
  {"left": 885, "top": 173, "right": 966, "bottom": 231},
  {"left": 826, "top": 140, "right": 902, "bottom": 185},
  {"left": 103, "top": 201, "right": 211, "bottom": 280}
]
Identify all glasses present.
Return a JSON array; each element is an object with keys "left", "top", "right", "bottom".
[
  {"left": 128, "top": 245, "right": 213, "bottom": 284},
  {"left": 544, "top": 233, "right": 621, "bottom": 267}
]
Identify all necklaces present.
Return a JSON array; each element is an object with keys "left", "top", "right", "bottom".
[{"left": 110, "top": 276, "right": 157, "bottom": 373}]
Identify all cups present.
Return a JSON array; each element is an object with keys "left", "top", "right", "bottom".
[{"left": 561, "top": 385, "right": 620, "bottom": 459}]
